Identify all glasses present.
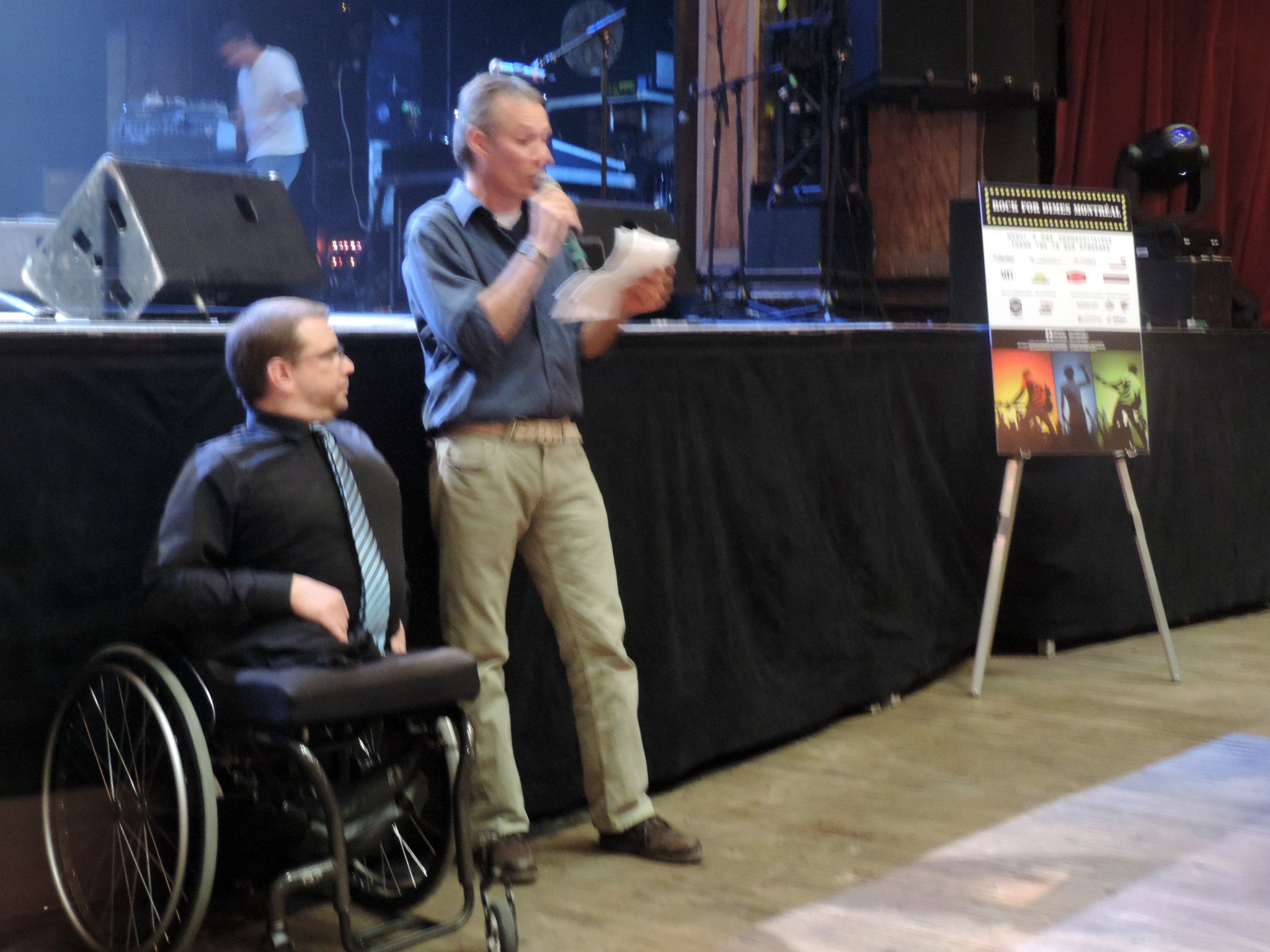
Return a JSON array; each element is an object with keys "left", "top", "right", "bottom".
[{"left": 280, "top": 343, "right": 347, "bottom": 370}]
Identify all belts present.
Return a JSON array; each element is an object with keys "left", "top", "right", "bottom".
[{"left": 451, "top": 418, "right": 583, "bottom": 445}]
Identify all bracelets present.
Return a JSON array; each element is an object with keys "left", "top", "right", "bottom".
[{"left": 515, "top": 241, "right": 556, "bottom": 268}]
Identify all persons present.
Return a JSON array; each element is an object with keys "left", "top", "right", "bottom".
[
  {"left": 217, "top": 20, "right": 313, "bottom": 189},
  {"left": 146, "top": 296, "right": 411, "bottom": 660},
  {"left": 1010, "top": 358, "right": 1143, "bottom": 437},
  {"left": 397, "top": 69, "right": 703, "bottom": 883}
]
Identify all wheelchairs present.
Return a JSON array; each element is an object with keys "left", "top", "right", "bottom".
[{"left": 33, "top": 555, "right": 532, "bottom": 952}]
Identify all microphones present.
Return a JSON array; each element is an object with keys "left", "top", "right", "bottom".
[
  {"left": 531, "top": 168, "right": 592, "bottom": 273},
  {"left": 488, "top": 57, "right": 558, "bottom": 87}
]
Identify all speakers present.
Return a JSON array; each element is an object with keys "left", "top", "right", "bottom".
[
  {"left": 19, "top": 155, "right": 311, "bottom": 324},
  {"left": 560, "top": 195, "right": 691, "bottom": 320}
]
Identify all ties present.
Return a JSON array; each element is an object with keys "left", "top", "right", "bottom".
[{"left": 309, "top": 421, "right": 391, "bottom": 656}]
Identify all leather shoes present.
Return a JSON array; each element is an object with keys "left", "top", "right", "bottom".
[
  {"left": 597, "top": 814, "right": 704, "bottom": 858},
  {"left": 471, "top": 836, "right": 541, "bottom": 884}
]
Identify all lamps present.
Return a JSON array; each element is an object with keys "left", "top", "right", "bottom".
[{"left": 1117, "top": 122, "right": 1220, "bottom": 238}]
